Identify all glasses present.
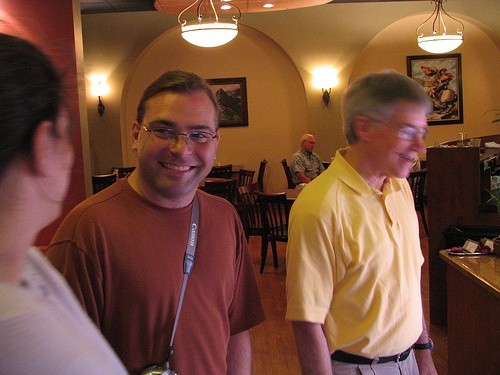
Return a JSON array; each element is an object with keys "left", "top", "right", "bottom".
[
  {"left": 367, "top": 117, "right": 429, "bottom": 141},
  {"left": 138, "top": 123, "right": 216, "bottom": 145}
]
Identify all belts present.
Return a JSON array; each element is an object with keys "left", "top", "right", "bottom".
[{"left": 328, "top": 345, "right": 413, "bottom": 365}]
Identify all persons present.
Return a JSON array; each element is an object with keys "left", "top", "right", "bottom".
[
  {"left": 0, "top": 33, "right": 130, "bottom": 374},
  {"left": 289, "top": 133, "right": 325, "bottom": 187},
  {"left": 285, "top": 70, "right": 439, "bottom": 375},
  {"left": 406, "top": 155, "right": 420, "bottom": 197},
  {"left": 45, "top": 70, "right": 265, "bottom": 375}
]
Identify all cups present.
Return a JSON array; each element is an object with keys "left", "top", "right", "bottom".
[
  {"left": 331, "top": 157, "right": 335, "bottom": 161},
  {"left": 433, "top": 142, "right": 441, "bottom": 148},
  {"left": 468, "top": 139, "right": 480, "bottom": 147}
]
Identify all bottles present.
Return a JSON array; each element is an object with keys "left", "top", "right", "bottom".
[{"left": 457, "top": 134, "right": 465, "bottom": 147}]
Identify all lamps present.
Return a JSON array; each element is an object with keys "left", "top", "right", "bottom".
[
  {"left": 311, "top": 67, "right": 338, "bottom": 107},
  {"left": 416, "top": 0, "right": 465, "bottom": 54},
  {"left": 178, "top": 0, "right": 242, "bottom": 48},
  {"left": 88, "top": 73, "right": 110, "bottom": 116}
]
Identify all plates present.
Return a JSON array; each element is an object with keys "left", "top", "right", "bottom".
[{"left": 446, "top": 247, "right": 496, "bottom": 255}]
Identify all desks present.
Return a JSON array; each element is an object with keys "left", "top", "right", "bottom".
[{"left": 438, "top": 249, "right": 500, "bottom": 375}]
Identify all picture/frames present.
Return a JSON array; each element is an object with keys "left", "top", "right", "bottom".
[
  {"left": 204, "top": 76, "right": 249, "bottom": 128},
  {"left": 405, "top": 52, "right": 464, "bottom": 127}
]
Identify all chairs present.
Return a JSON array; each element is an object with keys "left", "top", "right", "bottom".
[{"left": 90, "top": 158, "right": 429, "bottom": 274}]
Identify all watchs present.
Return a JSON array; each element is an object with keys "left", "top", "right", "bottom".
[{"left": 412, "top": 338, "right": 434, "bottom": 351}]
